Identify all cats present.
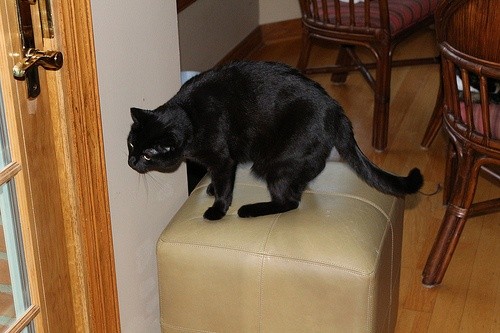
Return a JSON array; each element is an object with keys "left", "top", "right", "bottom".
[{"left": 127, "top": 61, "right": 425, "bottom": 220}]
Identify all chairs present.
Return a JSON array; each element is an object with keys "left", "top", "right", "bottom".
[
  {"left": 295, "top": 0, "right": 443, "bottom": 152},
  {"left": 421, "top": 0, "right": 500, "bottom": 288}
]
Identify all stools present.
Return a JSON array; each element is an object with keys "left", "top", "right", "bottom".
[{"left": 156, "top": 159, "right": 406, "bottom": 333}]
